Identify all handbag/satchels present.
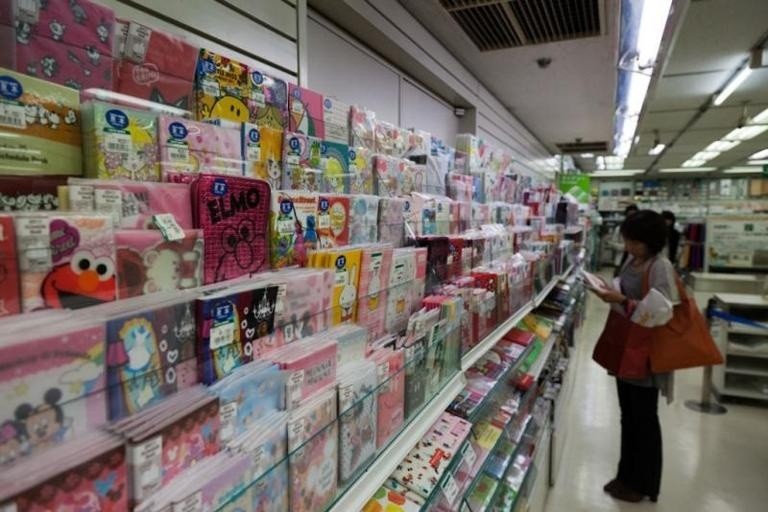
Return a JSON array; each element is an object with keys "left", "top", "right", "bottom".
[{"left": 592, "top": 298, "right": 724, "bottom": 380}]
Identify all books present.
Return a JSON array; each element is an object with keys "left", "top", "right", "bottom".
[{"left": 575, "top": 269, "right": 608, "bottom": 297}]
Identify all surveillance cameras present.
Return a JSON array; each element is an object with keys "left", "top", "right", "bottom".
[{"left": 538, "top": 57, "right": 552, "bottom": 69}]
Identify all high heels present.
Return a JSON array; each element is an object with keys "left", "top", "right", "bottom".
[{"left": 603, "top": 472, "right": 662, "bottom": 503}]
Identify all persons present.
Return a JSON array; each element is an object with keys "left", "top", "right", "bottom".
[
  {"left": 609, "top": 205, "right": 638, "bottom": 278},
  {"left": 660, "top": 210, "right": 680, "bottom": 266},
  {"left": 591, "top": 209, "right": 677, "bottom": 503}
]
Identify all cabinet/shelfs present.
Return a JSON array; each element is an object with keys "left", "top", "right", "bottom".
[
  {"left": 713, "top": 293, "right": 767, "bottom": 407},
  {"left": 0, "top": 65, "right": 596, "bottom": 511}
]
